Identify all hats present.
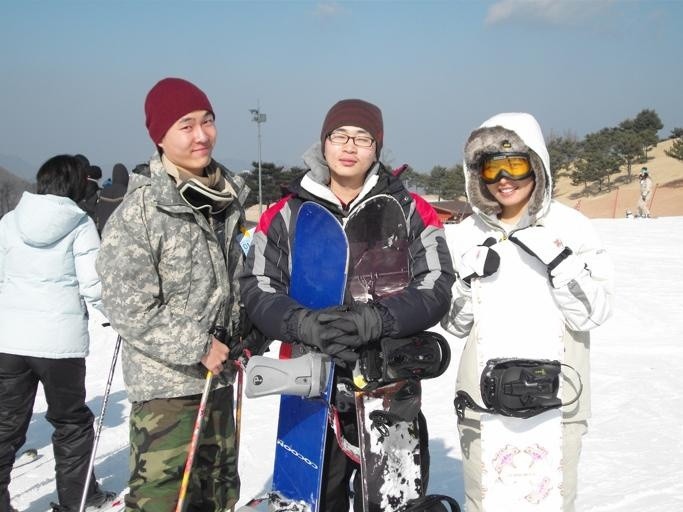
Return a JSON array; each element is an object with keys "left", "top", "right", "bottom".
[
  {"left": 641, "top": 167, "right": 649, "bottom": 174},
  {"left": 133, "top": 164, "right": 150, "bottom": 174},
  {"left": 320, "top": 99, "right": 383, "bottom": 162},
  {"left": 145, "top": 78, "right": 216, "bottom": 146}
]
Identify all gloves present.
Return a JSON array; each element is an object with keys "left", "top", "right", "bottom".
[
  {"left": 228, "top": 328, "right": 267, "bottom": 374},
  {"left": 509, "top": 225, "right": 587, "bottom": 288},
  {"left": 198, "top": 326, "right": 234, "bottom": 385},
  {"left": 642, "top": 195, "right": 646, "bottom": 201},
  {"left": 318, "top": 302, "right": 383, "bottom": 356},
  {"left": 298, "top": 306, "right": 359, "bottom": 361},
  {"left": 457, "top": 237, "right": 500, "bottom": 291},
  {"left": 639, "top": 174, "right": 643, "bottom": 180}
]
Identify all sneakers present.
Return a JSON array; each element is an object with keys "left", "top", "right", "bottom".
[{"left": 57, "top": 491, "right": 124, "bottom": 512}]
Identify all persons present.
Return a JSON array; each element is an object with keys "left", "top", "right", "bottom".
[
  {"left": 0, "top": 154, "right": 117, "bottom": 512},
  {"left": 638, "top": 174, "right": 646, "bottom": 217},
  {"left": 87, "top": 164, "right": 108, "bottom": 201},
  {"left": 74, "top": 154, "right": 97, "bottom": 225},
  {"left": 441, "top": 113, "right": 615, "bottom": 512},
  {"left": 95, "top": 77, "right": 269, "bottom": 512},
  {"left": 639, "top": 167, "right": 652, "bottom": 218},
  {"left": 102, "top": 178, "right": 112, "bottom": 190},
  {"left": 95, "top": 164, "right": 129, "bottom": 240},
  {"left": 238, "top": 99, "right": 455, "bottom": 512}
]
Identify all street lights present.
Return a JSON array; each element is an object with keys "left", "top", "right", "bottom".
[{"left": 248, "top": 109, "right": 267, "bottom": 226}]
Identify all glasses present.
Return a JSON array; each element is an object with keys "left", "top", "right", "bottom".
[
  {"left": 327, "top": 133, "right": 376, "bottom": 147},
  {"left": 479, "top": 152, "right": 533, "bottom": 184},
  {"left": 160, "top": 153, "right": 235, "bottom": 215}
]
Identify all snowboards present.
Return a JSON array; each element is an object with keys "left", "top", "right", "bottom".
[
  {"left": 344, "top": 193, "right": 462, "bottom": 512},
  {"left": 243, "top": 199, "right": 350, "bottom": 512},
  {"left": 46, "top": 487, "right": 131, "bottom": 512},
  {"left": 232, "top": 497, "right": 270, "bottom": 512}
]
